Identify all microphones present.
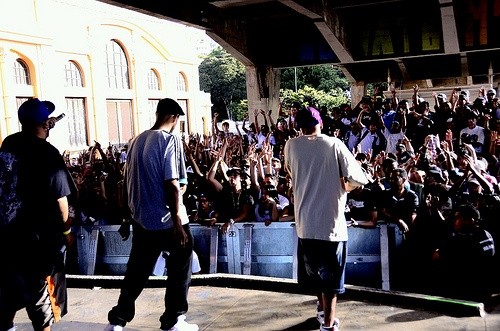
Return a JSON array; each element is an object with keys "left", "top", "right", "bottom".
[{"left": 55, "top": 113, "right": 66, "bottom": 122}]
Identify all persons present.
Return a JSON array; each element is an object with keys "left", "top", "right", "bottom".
[
  {"left": 104, "top": 98, "right": 200, "bottom": 331},
  {"left": 62, "top": 84, "right": 500, "bottom": 306},
  {"left": 284, "top": 108, "right": 369, "bottom": 331},
  {"left": 0, "top": 98, "right": 78, "bottom": 331}
]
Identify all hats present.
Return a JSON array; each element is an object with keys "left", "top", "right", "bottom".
[
  {"left": 295, "top": 107, "right": 322, "bottom": 127},
  {"left": 486, "top": 89, "right": 496, "bottom": 95},
  {"left": 156, "top": 98, "right": 185, "bottom": 116},
  {"left": 18, "top": 98, "right": 55, "bottom": 125}
]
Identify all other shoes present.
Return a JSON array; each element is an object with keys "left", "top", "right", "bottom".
[
  {"left": 320, "top": 318, "right": 339, "bottom": 331},
  {"left": 160, "top": 315, "right": 199, "bottom": 331},
  {"left": 316, "top": 311, "right": 324, "bottom": 324},
  {"left": 104, "top": 322, "right": 124, "bottom": 331}
]
